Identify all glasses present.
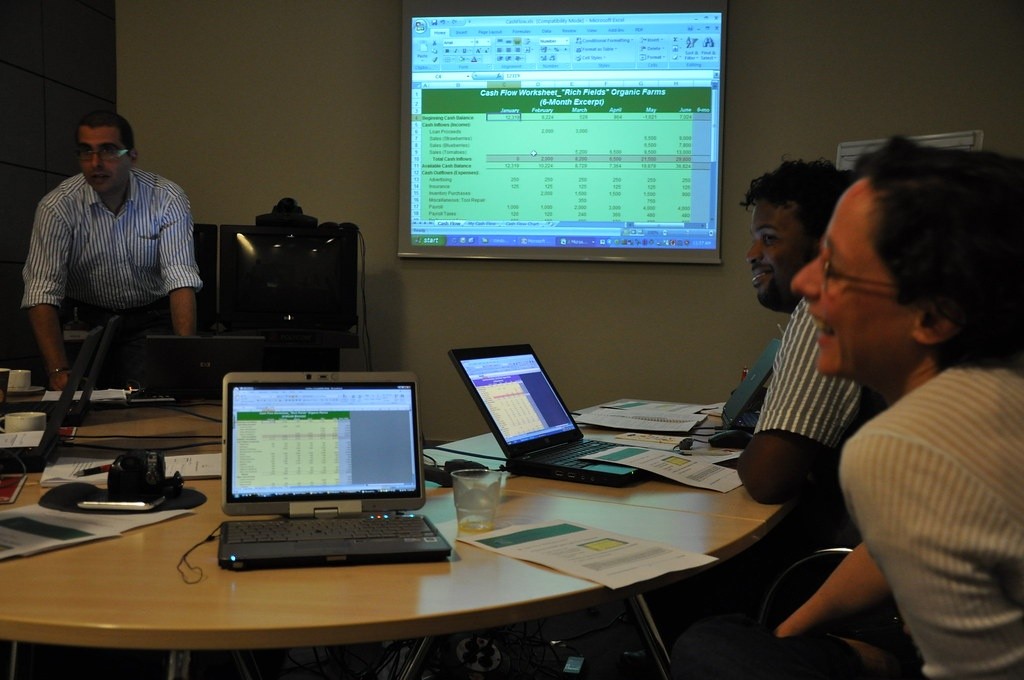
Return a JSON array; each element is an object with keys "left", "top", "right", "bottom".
[
  {"left": 73, "top": 145, "right": 132, "bottom": 160},
  {"left": 816, "top": 235, "right": 900, "bottom": 296}
]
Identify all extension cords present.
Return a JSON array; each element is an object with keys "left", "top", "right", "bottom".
[{"left": 456, "top": 638, "right": 501, "bottom": 671}]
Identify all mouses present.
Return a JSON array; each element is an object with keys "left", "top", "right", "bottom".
[{"left": 708, "top": 430, "right": 753, "bottom": 449}]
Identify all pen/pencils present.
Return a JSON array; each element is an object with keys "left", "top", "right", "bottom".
[
  {"left": 741, "top": 365, "right": 748, "bottom": 381},
  {"left": 73, "top": 464, "right": 113, "bottom": 476}
]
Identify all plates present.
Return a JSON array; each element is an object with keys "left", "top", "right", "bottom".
[{"left": 7, "top": 386, "right": 44, "bottom": 396}]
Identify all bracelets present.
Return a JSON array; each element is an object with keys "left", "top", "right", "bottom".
[{"left": 48, "top": 366, "right": 72, "bottom": 377}]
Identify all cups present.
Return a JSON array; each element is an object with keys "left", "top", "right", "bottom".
[
  {"left": 0, "top": 368, "right": 10, "bottom": 406},
  {"left": 8, "top": 370, "right": 31, "bottom": 390},
  {"left": 450, "top": 468, "right": 502, "bottom": 533},
  {"left": 0, "top": 412, "right": 47, "bottom": 433}
]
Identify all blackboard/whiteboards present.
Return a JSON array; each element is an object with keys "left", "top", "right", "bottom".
[{"left": 835, "top": 130, "right": 983, "bottom": 169}]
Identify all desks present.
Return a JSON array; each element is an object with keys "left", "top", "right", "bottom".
[{"left": 0, "top": 364, "right": 789, "bottom": 679}]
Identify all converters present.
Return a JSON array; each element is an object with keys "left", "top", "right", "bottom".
[{"left": 562, "top": 657, "right": 585, "bottom": 676}]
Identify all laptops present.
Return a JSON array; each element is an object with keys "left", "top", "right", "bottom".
[
  {"left": 218, "top": 372, "right": 451, "bottom": 571},
  {"left": 447, "top": 343, "right": 652, "bottom": 487},
  {"left": 0, "top": 316, "right": 123, "bottom": 475},
  {"left": 722, "top": 338, "right": 786, "bottom": 433},
  {"left": 127, "top": 334, "right": 267, "bottom": 406}
]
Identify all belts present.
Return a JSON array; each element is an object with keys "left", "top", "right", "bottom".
[{"left": 64, "top": 296, "right": 168, "bottom": 315}]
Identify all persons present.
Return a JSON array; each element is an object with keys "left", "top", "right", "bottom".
[
  {"left": 775, "top": 135, "right": 1024, "bottom": 680},
  {"left": 736, "top": 158, "right": 905, "bottom": 503},
  {"left": 20, "top": 112, "right": 204, "bottom": 390}
]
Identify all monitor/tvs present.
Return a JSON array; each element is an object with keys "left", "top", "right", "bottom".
[{"left": 217, "top": 224, "right": 357, "bottom": 334}]
